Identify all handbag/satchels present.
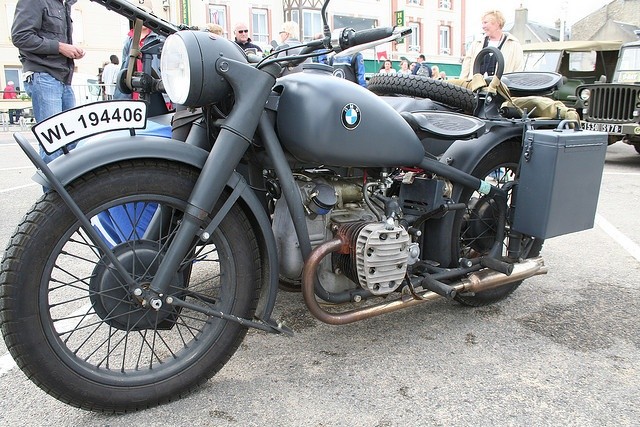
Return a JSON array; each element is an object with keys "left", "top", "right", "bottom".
[{"left": 416, "top": 63, "right": 429, "bottom": 76}]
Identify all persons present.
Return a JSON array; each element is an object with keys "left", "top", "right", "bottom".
[
  {"left": 4, "top": 81, "right": 21, "bottom": 125},
  {"left": 379, "top": 60, "right": 396, "bottom": 75},
  {"left": 113, "top": 5, "right": 175, "bottom": 112},
  {"left": 202, "top": 23, "right": 224, "bottom": 38},
  {"left": 412, "top": 55, "right": 432, "bottom": 77},
  {"left": 97, "top": 61, "right": 110, "bottom": 101},
  {"left": 15, "top": 87, "right": 20, "bottom": 98},
  {"left": 275, "top": 22, "right": 304, "bottom": 58},
  {"left": 410, "top": 62, "right": 417, "bottom": 73},
  {"left": 233, "top": 22, "right": 263, "bottom": 52},
  {"left": 438, "top": 71, "right": 446, "bottom": 81},
  {"left": 431, "top": 65, "right": 440, "bottom": 81},
  {"left": 458, "top": 10, "right": 524, "bottom": 81},
  {"left": 397, "top": 60, "right": 412, "bottom": 75},
  {"left": 102, "top": 55, "right": 120, "bottom": 96},
  {"left": 329, "top": 51, "right": 367, "bottom": 87},
  {"left": 311, "top": 33, "right": 329, "bottom": 65},
  {"left": 10, "top": 0, "right": 86, "bottom": 193}
]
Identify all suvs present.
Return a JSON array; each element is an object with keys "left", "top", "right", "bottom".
[
  {"left": 574, "top": 42, "right": 640, "bottom": 153},
  {"left": 523, "top": 40, "right": 622, "bottom": 109}
]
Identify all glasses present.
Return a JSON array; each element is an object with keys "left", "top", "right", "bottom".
[{"left": 239, "top": 30, "right": 248, "bottom": 33}]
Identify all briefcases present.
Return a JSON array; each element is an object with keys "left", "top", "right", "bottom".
[{"left": 511, "top": 120, "right": 608, "bottom": 239}]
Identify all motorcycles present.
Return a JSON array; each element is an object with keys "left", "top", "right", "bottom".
[{"left": 0, "top": 0, "right": 608, "bottom": 415}]
